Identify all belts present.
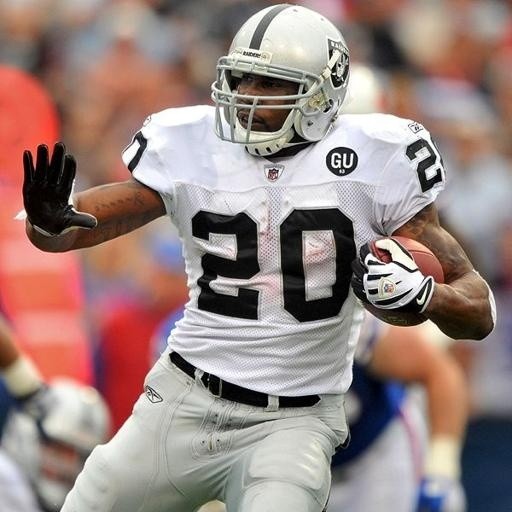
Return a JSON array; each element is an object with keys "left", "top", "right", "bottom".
[{"left": 167, "top": 353, "right": 318, "bottom": 408}]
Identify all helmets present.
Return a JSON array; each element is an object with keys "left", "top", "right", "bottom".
[
  {"left": 213, "top": 3, "right": 350, "bottom": 157},
  {"left": 24, "top": 373, "right": 111, "bottom": 511}
]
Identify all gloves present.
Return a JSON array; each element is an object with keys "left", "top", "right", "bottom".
[
  {"left": 416, "top": 478, "right": 473, "bottom": 512},
  {"left": 350, "top": 237, "right": 433, "bottom": 316},
  {"left": 22, "top": 141, "right": 98, "bottom": 239}
]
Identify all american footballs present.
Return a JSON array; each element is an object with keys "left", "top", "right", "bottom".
[{"left": 356, "top": 235, "right": 444, "bottom": 326}]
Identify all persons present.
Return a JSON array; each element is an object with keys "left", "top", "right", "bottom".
[
  {"left": 324, "top": 311, "right": 468, "bottom": 512},
  {"left": 21, "top": 4, "right": 497, "bottom": 512},
  {"left": 0, "top": 0, "right": 512, "bottom": 407},
  {"left": 0, "top": 324, "right": 111, "bottom": 512}
]
